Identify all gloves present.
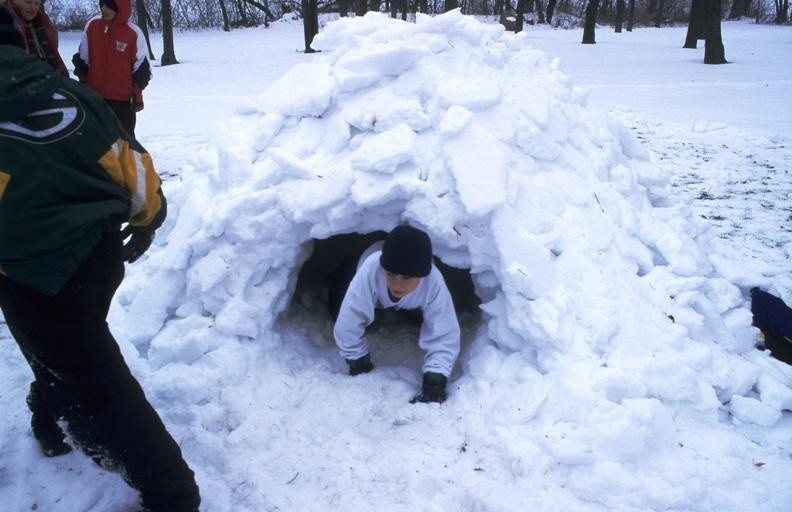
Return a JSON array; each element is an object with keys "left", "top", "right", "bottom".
[
  {"left": 345, "top": 353, "right": 374, "bottom": 377},
  {"left": 120, "top": 223, "right": 155, "bottom": 264},
  {"left": 408, "top": 372, "right": 448, "bottom": 404}
]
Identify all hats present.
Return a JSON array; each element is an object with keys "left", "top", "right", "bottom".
[{"left": 380, "top": 225, "right": 432, "bottom": 277}]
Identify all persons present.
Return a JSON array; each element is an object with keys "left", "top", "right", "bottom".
[
  {"left": 0, "top": 44, "right": 204, "bottom": 512},
  {"left": 1, "top": 1, "right": 71, "bottom": 80},
  {"left": 71, "top": 2, "right": 153, "bottom": 137},
  {"left": 331, "top": 224, "right": 461, "bottom": 403}
]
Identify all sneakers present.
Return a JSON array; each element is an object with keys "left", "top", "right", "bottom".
[{"left": 31, "top": 413, "right": 72, "bottom": 457}]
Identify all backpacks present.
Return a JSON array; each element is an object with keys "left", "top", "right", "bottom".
[{"left": 750, "top": 286, "right": 792, "bottom": 366}]
